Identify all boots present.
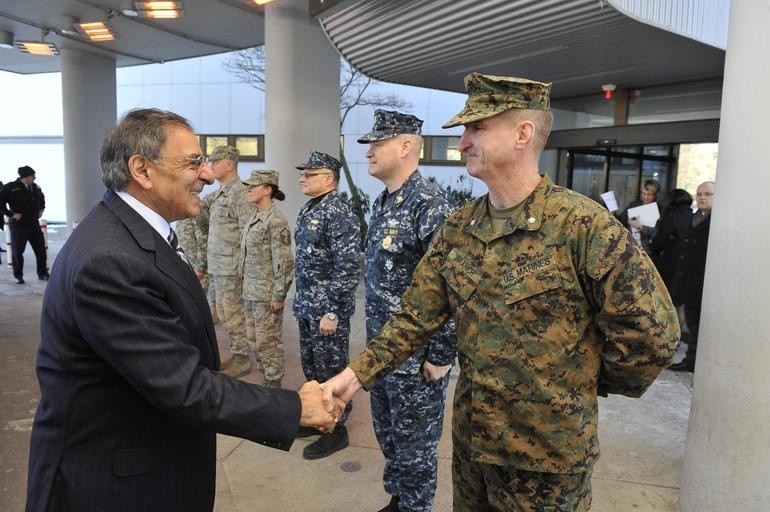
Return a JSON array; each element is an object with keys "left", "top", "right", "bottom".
[
  {"left": 297, "top": 426, "right": 320, "bottom": 437},
  {"left": 220, "top": 355, "right": 236, "bottom": 369},
  {"left": 263, "top": 379, "right": 281, "bottom": 388},
  {"left": 221, "top": 358, "right": 252, "bottom": 379},
  {"left": 303, "top": 424, "right": 349, "bottom": 460}
]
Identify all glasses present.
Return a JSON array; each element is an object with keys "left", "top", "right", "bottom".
[
  {"left": 300, "top": 172, "right": 330, "bottom": 179},
  {"left": 145, "top": 154, "right": 209, "bottom": 171}
]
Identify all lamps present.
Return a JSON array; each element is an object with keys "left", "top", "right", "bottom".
[
  {"left": 72, "top": 18, "right": 118, "bottom": 43},
  {"left": 246, "top": 0, "right": 273, "bottom": 10},
  {"left": 602, "top": 83, "right": 618, "bottom": 101},
  {"left": 136, "top": 0, "right": 184, "bottom": 20},
  {"left": 12, "top": 40, "right": 60, "bottom": 57},
  {"left": 630, "top": 90, "right": 641, "bottom": 105}
]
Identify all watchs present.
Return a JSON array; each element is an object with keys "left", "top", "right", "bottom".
[{"left": 325, "top": 312, "right": 338, "bottom": 321}]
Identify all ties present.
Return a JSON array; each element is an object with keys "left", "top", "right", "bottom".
[{"left": 167, "top": 227, "right": 198, "bottom": 282}]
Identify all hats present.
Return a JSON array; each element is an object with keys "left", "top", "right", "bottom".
[
  {"left": 18, "top": 166, "right": 35, "bottom": 177},
  {"left": 442, "top": 72, "right": 552, "bottom": 129},
  {"left": 357, "top": 110, "right": 424, "bottom": 144},
  {"left": 208, "top": 146, "right": 239, "bottom": 163},
  {"left": 241, "top": 170, "right": 279, "bottom": 186},
  {"left": 295, "top": 152, "right": 342, "bottom": 170}
]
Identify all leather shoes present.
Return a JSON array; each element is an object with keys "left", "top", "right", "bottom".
[
  {"left": 15, "top": 277, "right": 25, "bottom": 284},
  {"left": 39, "top": 275, "right": 50, "bottom": 281},
  {"left": 667, "top": 360, "right": 695, "bottom": 373}
]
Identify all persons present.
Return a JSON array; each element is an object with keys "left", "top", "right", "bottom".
[
  {"left": 2, "top": 166, "right": 51, "bottom": 284},
  {"left": 295, "top": 151, "right": 360, "bottom": 460},
  {"left": 0, "top": 181, "right": 6, "bottom": 265},
  {"left": 612, "top": 179, "right": 661, "bottom": 228},
  {"left": 197, "top": 185, "right": 218, "bottom": 324},
  {"left": 241, "top": 169, "right": 293, "bottom": 388},
  {"left": 321, "top": 73, "right": 682, "bottom": 512},
  {"left": 25, "top": 109, "right": 344, "bottom": 512},
  {"left": 357, "top": 109, "right": 455, "bottom": 512},
  {"left": 628, "top": 189, "right": 694, "bottom": 327},
  {"left": 171, "top": 218, "right": 203, "bottom": 273},
  {"left": 206, "top": 147, "right": 257, "bottom": 380},
  {"left": 667, "top": 182, "right": 715, "bottom": 373}
]
